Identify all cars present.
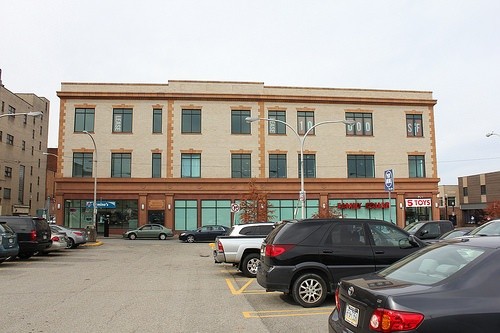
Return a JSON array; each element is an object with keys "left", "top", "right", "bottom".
[
  {"left": 123, "top": 223, "right": 175, "bottom": 240},
  {"left": 178, "top": 224, "right": 231, "bottom": 243},
  {"left": 326, "top": 218, "right": 500, "bottom": 333},
  {"left": 40, "top": 223, "right": 88, "bottom": 253}
]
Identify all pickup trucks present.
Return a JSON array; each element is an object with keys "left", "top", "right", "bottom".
[{"left": 212, "top": 220, "right": 283, "bottom": 279}]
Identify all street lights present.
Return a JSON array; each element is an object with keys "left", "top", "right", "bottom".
[
  {"left": 83, "top": 130, "right": 97, "bottom": 229},
  {"left": 244, "top": 116, "right": 356, "bottom": 219}
]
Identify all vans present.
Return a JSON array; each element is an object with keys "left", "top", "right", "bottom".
[{"left": 394, "top": 219, "right": 454, "bottom": 242}]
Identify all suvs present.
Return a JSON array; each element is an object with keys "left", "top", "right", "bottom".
[
  {"left": 0, "top": 215, "right": 54, "bottom": 259},
  {"left": 255, "top": 217, "right": 434, "bottom": 309},
  {"left": 225, "top": 220, "right": 282, "bottom": 238},
  {"left": 0, "top": 223, "right": 20, "bottom": 264}
]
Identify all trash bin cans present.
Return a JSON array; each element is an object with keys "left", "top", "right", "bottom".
[{"left": 86, "top": 225, "right": 97, "bottom": 242}]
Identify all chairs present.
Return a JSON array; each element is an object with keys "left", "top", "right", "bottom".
[{"left": 343, "top": 231, "right": 364, "bottom": 244}]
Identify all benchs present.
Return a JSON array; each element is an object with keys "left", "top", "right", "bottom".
[{"left": 415, "top": 258, "right": 467, "bottom": 279}]
